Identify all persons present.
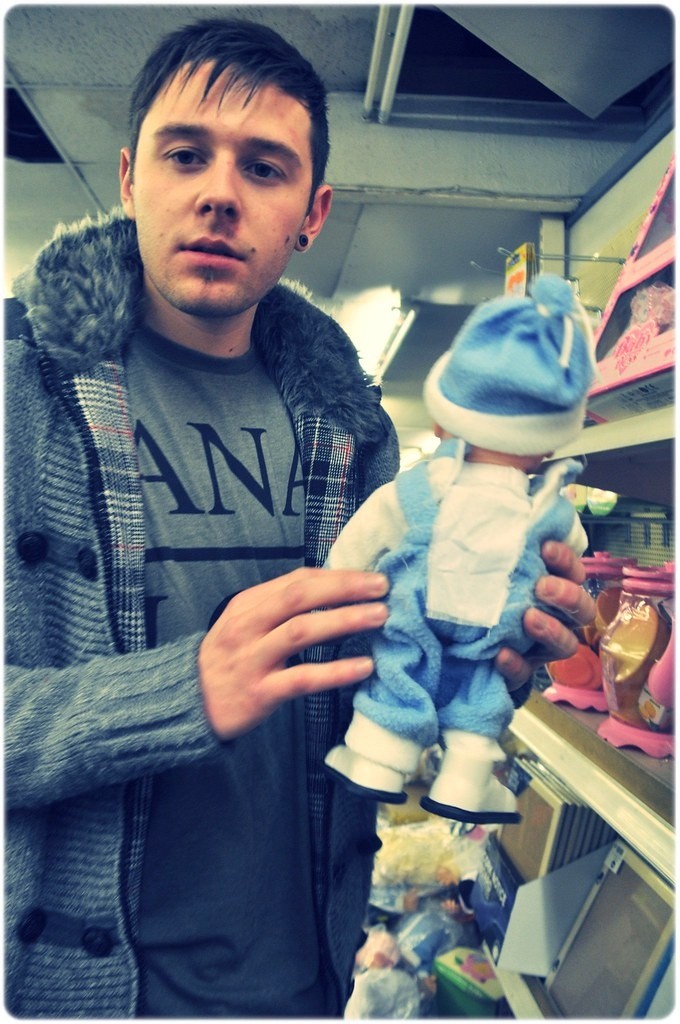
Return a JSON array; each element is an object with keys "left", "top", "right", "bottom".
[
  {"left": 4, "top": 17, "right": 596, "bottom": 1019},
  {"left": 324, "top": 275, "right": 595, "bottom": 825}
]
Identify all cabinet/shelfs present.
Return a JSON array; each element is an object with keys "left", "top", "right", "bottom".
[{"left": 435, "top": 406, "right": 677, "bottom": 1020}]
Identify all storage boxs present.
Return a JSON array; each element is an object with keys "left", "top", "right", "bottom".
[{"left": 583, "top": 149, "right": 676, "bottom": 423}]
